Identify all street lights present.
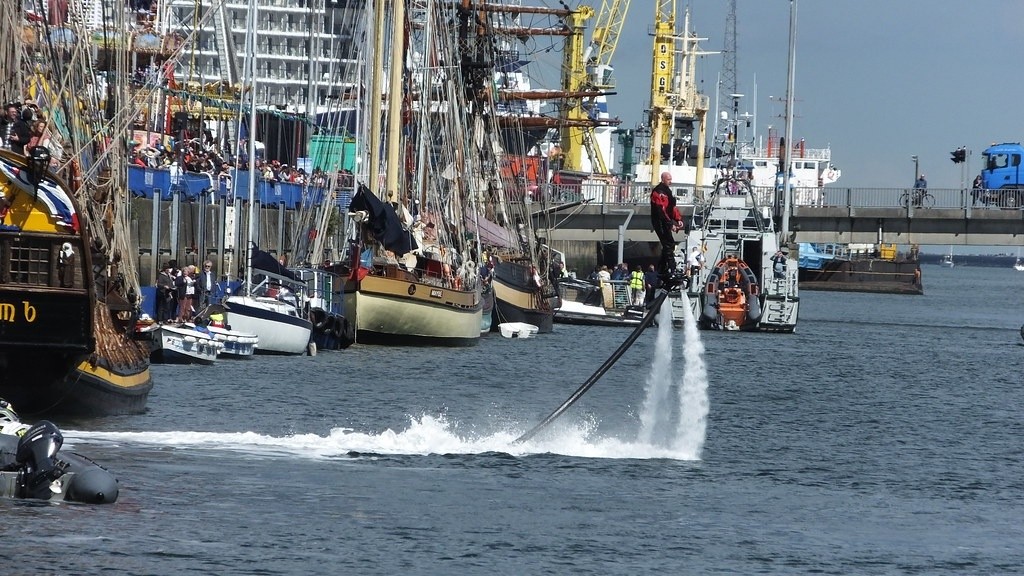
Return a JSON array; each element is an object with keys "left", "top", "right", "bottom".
[{"left": 910, "top": 154, "right": 919, "bottom": 207}]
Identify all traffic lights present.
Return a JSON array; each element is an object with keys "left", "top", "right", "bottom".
[
  {"left": 950, "top": 149, "right": 960, "bottom": 164},
  {"left": 958, "top": 149, "right": 966, "bottom": 162}
]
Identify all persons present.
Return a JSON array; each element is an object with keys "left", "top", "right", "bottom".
[
  {"left": 134, "top": 64, "right": 170, "bottom": 87},
  {"left": 913, "top": 174, "right": 928, "bottom": 209},
  {"left": 650, "top": 172, "right": 683, "bottom": 273},
  {"left": 264, "top": 281, "right": 279, "bottom": 299},
  {"left": 990, "top": 156, "right": 1007, "bottom": 167},
  {"left": 726, "top": 174, "right": 750, "bottom": 195},
  {"left": 127, "top": 119, "right": 353, "bottom": 188},
  {"left": 0, "top": 100, "right": 59, "bottom": 174},
  {"left": 770, "top": 251, "right": 787, "bottom": 279},
  {"left": 687, "top": 246, "right": 704, "bottom": 285},
  {"left": 549, "top": 262, "right": 660, "bottom": 308},
  {"left": 972, "top": 175, "right": 982, "bottom": 207},
  {"left": 157, "top": 260, "right": 215, "bottom": 326}
]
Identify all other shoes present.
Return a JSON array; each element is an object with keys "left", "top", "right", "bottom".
[
  {"left": 158, "top": 318, "right": 192, "bottom": 325},
  {"left": 672, "top": 269, "right": 683, "bottom": 275},
  {"left": 658, "top": 268, "right": 670, "bottom": 274}
]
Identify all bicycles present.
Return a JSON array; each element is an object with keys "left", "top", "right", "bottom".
[{"left": 898, "top": 189, "right": 936, "bottom": 208}]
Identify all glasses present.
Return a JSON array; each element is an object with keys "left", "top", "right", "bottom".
[{"left": 205, "top": 265, "right": 212, "bottom": 268}]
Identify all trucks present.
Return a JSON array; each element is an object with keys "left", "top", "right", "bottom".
[{"left": 979, "top": 142, "right": 1024, "bottom": 210}]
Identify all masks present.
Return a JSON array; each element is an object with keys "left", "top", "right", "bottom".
[{"left": 167, "top": 268, "right": 172, "bottom": 273}]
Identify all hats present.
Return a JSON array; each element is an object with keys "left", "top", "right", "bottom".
[{"left": 160, "top": 262, "right": 170, "bottom": 272}]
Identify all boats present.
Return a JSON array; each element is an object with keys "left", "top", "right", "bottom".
[{"left": 1, "top": 0, "right": 922, "bottom": 505}]
